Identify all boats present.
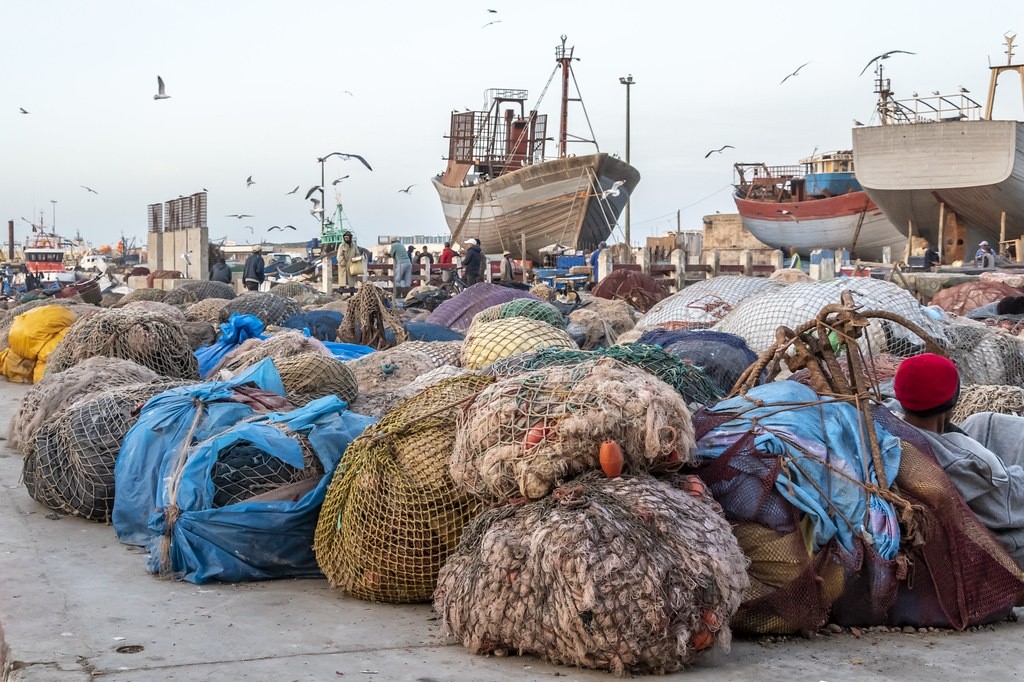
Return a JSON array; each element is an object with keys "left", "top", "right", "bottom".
[
  {"left": 13, "top": 211, "right": 76, "bottom": 287},
  {"left": 732, "top": 150, "right": 910, "bottom": 262},
  {"left": 852, "top": 29, "right": 1024, "bottom": 259},
  {"left": 803, "top": 152, "right": 861, "bottom": 197},
  {"left": 433, "top": 36, "right": 641, "bottom": 265},
  {"left": 277, "top": 184, "right": 372, "bottom": 283},
  {"left": 80, "top": 255, "right": 118, "bottom": 272},
  {"left": 264, "top": 252, "right": 293, "bottom": 280}
]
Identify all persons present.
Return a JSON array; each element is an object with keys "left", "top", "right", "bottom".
[
  {"left": 462, "top": 238, "right": 487, "bottom": 287},
  {"left": 440, "top": 242, "right": 455, "bottom": 264},
  {"left": 209, "top": 258, "right": 232, "bottom": 284},
  {"left": 385, "top": 235, "right": 412, "bottom": 286},
  {"left": 590, "top": 241, "right": 606, "bottom": 282},
  {"left": 242, "top": 245, "right": 265, "bottom": 291},
  {"left": 895, "top": 353, "right": 1024, "bottom": 571},
  {"left": 976, "top": 241, "right": 997, "bottom": 267},
  {"left": 790, "top": 247, "right": 802, "bottom": 269},
  {"left": 500, "top": 251, "right": 514, "bottom": 281},
  {"left": 922, "top": 243, "right": 940, "bottom": 273},
  {"left": 407, "top": 245, "right": 435, "bottom": 275},
  {"left": 336, "top": 231, "right": 360, "bottom": 296}
]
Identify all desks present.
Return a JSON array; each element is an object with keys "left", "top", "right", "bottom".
[{"left": 554, "top": 276, "right": 587, "bottom": 293}]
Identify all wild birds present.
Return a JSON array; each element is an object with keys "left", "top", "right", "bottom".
[
  {"left": 317, "top": 153, "right": 373, "bottom": 172},
  {"left": 781, "top": 62, "right": 809, "bottom": 84},
  {"left": 481, "top": 9, "right": 501, "bottom": 27},
  {"left": 286, "top": 175, "right": 348, "bottom": 222},
  {"left": 226, "top": 214, "right": 254, "bottom": 219},
  {"left": 19, "top": 107, "right": 31, "bottom": 114},
  {"left": 600, "top": 180, "right": 626, "bottom": 200},
  {"left": 182, "top": 251, "right": 194, "bottom": 265},
  {"left": 859, "top": 50, "right": 916, "bottom": 77},
  {"left": 345, "top": 91, "right": 352, "bottom": 96},
  {"left": 246, "top": 175, "right": 256, "bottom": 187},
  {"left": 154, "top": 76, "right": 171, "bottom": 100},
  {"left": 777, "top": 209, "right": 798, "bottom": 224},
  {"left": 398, "top": 185, "right": 414, "bottom": 192},
  {"left": 268, "top": 226, "right": 296, "bottom": 232},
  {"left": 852, "top": 119, "right": 864, "bottom": 128},
  {"left": 82, "top": 186, "right": 97, "bottom": 194},
  {"left": 244, "top": 226, "right": 254, "bottom": 234},
  {"left": 912, "top": 85, "right": 970, "bottom": 96},
  {"left": 705, "top": 145, "right": 734, "bottom": 159}
]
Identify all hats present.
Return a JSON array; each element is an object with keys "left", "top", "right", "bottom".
[
  {"left": 503, "top": 250, "right": 510, "bottom": 256},
  {"left": 894, "top": 352, "right": 960, "bottom": 416},
  {"left": 252, "top": 245, "right": 265, "bottom": 252},
  {"left": 343, "top": 231, "right": 353, "bottom": 236},
  {"left": 979, "top": 241, "right": 988, "bottom": 246},
  {"left": 464, "top": 238, "right": 477, "bottom": 245},
  {"left": 390, "top": 236, "right": 396, "bottom": 242},
  {"left": 409, "top": 245, "right": 416, "bottom": 249}
]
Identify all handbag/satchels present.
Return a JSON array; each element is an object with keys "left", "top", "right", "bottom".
[{"left": 350, "top": 260, "right": 365, "bottom": 275}]
